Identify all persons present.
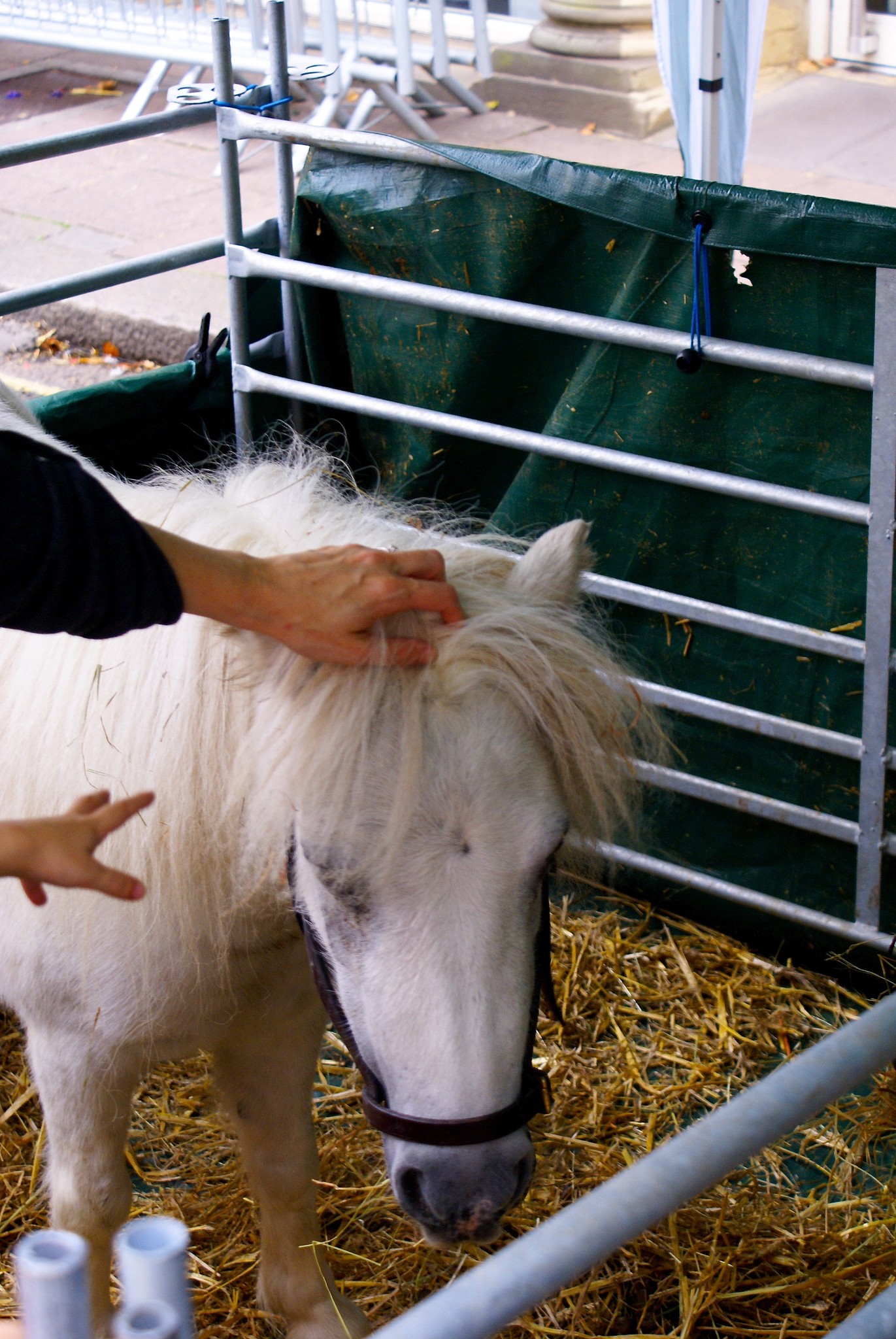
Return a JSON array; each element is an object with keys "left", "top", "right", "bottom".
[
  {"left": 0, "top": 791, "right": 155, "bottom": 907},
  {"left": 0, "top": 426, "right": 466, "bottom": 669}
]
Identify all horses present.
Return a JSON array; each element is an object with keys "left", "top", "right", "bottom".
[{"left": 0, "top": 405, "right": 683, "bottom": 1339}]
